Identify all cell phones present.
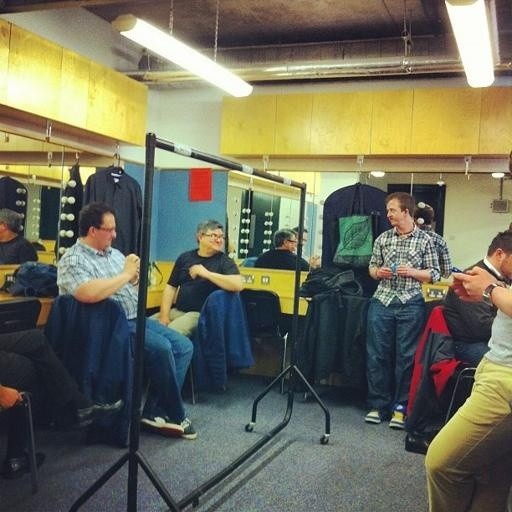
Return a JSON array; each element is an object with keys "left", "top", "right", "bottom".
[
  {"left": 453, "top": 267, "right": 464, "bottom": 273},
  {"left": 390, "top": 263, "right": 398, "bottom": 272}
]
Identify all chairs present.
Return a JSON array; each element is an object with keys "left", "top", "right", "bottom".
[
  {"left": 189, "top": 290, "right": 254, "bottom": 405},
  {"left": 20, "top": 391, "right": 39, "bottom": 493},
  {"left": 435, "top": 305, "right": 478, "bottom": 428},
  {"left": 0, "top": 297, "right": 42, "bottom": 332},
  {"left": 238, "top": 288, "right": 288, "bottom": 394}
]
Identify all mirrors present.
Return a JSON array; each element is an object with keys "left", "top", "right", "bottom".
[
  {"left": 226, "top": 170, "right": 511, "bottom": 283},
  {"left": 0, "top": 131, "right": 123, "bottom": 264}
]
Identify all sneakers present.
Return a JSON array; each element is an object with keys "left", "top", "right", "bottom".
[
  {"left": 389, "top": 404, "right": 407, "bottom": 430},
  {"left": 182, "top": 419, "right": 197, "bottom": 440},
  {"left": 142, "top": 415, "right": 183, "bottom": 433},
  {"left": 364, "top": 409, "right": 384, "bottom": 423}
]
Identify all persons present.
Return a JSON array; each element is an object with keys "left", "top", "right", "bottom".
[
  {"left": 149, "top": 220, "right": 243, "bottom": 338},
  {"left": 254, "top": 228, "right": 314, "bottom": 271},
  {"left": 364, "top": 191, "right": 442, "bottom": 430},
  {"left": 0, "top": 328, "right": 125, "bottom": 477},
  {"left": 293, "top": 227, "right": 320, "bottom": 269},
  {"left": 442, "top": 223, "right": 512, "bottom": 369},
  {"left": 413, "top": 203, "right": 452, "bottom": 279},
  {"left": 55, "top": 201, "right": 199, "bottom": 440},
  {"left": 424, "top": 265, "right": 512, "bottom": 512},
  {"left": 0, "top": 208, "right": 39, "bottom": 265}
]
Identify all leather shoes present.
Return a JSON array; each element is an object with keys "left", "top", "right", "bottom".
[
  {"left": 5, "top": 453, "right": 44, "bottom": 479},
  {"left": 74, "top": 399, "right": 123, "bottom": 428}
]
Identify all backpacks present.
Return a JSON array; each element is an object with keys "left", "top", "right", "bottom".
[
  {"left": 298, "top": 266, "right": 363, "bottom": 298},
  {"left": 5, "top": 260, "right": 59, "bottom": 299}
]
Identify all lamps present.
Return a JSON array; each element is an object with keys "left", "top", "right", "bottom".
[
  {"left": 109, "top": 0, "right": 254, "bottom": 98},
  {"left": 444, "top": 0, "right": 501, "bottom": 89}
]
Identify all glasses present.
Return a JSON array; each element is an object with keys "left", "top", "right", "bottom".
[
  {"left": 203, "top": 234, "right": 223, "bottom": 240},
  {"left": 97, "top": 226, "right": 116, "bottom": 234}
]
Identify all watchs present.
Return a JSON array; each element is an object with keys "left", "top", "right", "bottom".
[{"left": 482, "top": 283, "right": 499, "bottom": 308}]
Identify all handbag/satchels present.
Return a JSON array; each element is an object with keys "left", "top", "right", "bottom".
[{"left": 333, "top": 214, "right": 374, "bottom": 269}]
